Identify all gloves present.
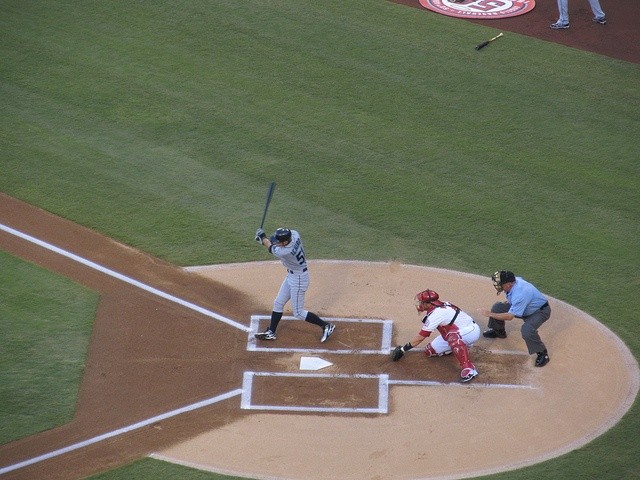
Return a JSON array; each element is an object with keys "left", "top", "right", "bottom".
[
  {"left": 255, "top": 236, "right": 262, "bottom": 245},
  {"left": 256, "top": 229, "right": 266, "bottom": 239}
]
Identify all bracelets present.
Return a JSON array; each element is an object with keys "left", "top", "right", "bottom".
[{"left": 403, "top": 342, "right": 413, "bottom": 351}]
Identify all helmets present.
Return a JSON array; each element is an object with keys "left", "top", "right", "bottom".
[
  {"left": 492, "top": 271, "right": 515, "bottom": 296},
  {"left": 414, "top": 290, "right": 444, "bottom": 312},
  {"left": 270, "top": 228, "right": 291, "bottom": 243}
]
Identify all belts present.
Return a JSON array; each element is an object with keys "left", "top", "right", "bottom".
[
  {"left": 472, "top": 320, "right": 475, "bottom": 324},
  {"left": 541, "top": 302, "right": 547, "bottom": 310},
  {"left": 287, "top": 268, "right": 307, "bottom": 274}
]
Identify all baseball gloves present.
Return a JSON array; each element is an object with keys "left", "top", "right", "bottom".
[{"left": 390, "top": 345, "right": 405, "bottom": 361}]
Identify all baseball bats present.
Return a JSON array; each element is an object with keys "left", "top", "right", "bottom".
[
  {"left": 256, "top": 182, "right": 276, "bottom": 241},
  {"left": 475, "top": 32, "right": 504, "bottom": 51}
]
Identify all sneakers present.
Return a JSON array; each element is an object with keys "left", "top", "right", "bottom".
[
  {"left": 592, "top": 16, "right": 607, "bottom": 24},
  {"left": 320, "top": 322, "right": 336, "bottom": 343},
  {"left": 535, "top": 354, "right": 549, "bottom": 367},
  {"left": 255, "top": 330, "right": 277, "bottom": 340},
  {"left": 549, "top": 22, "right": 570, "bottom": 29},
  {"left": 460, "top": 368, "right": 479, "bottom": 383},
  {"left": 483, "top": 330, "right": 506, "bottom": 339}
]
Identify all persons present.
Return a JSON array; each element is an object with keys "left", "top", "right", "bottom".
[
  {"left": 392, "top": 290, "right": 481, "bottom": 383},
  {"left": 481, "top": 271, "right": 551, "bottom": 367},
  {"left": 549, "top": 0, "right": 607, "bottom": 29},
  {"left": 255, "top": 228, "right": 336, "bottom": 343}
]
